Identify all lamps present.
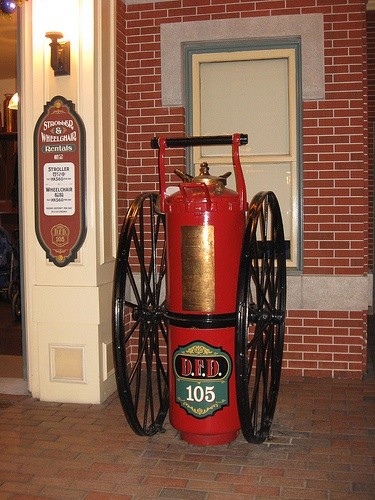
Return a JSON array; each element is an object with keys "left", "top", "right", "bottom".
[{"left": 43, "top": 1, "right": 72, "bottom": 76}]
